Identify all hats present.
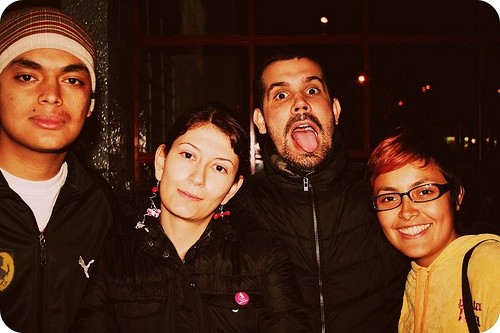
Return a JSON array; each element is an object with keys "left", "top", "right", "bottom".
[{"left": 0, "top": 0, "right": 97, "bottom": 111}]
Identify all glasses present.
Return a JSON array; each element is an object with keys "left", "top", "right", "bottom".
[{"left": 366, "top": 182, "right": 459, "bottom": 213}]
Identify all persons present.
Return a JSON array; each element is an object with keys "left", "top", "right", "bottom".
[
  {"left": 0, "top": 8, "right": 123, "bottom": 333},
  {"left": 79, "top": 107, "right": 311, "bottom": 332},
  {"left": 247, "top": 49, "right": 411, "bottom": 332},
  {"left": 365, "top": 127, "right": 500, "bottom": 332}
]
